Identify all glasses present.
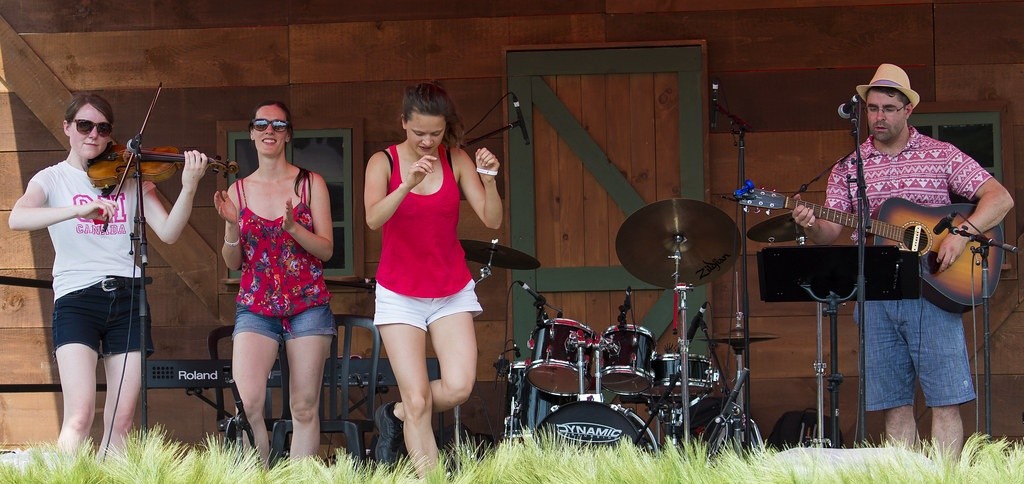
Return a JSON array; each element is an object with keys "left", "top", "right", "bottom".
[
  {"left": 67, "top": 119, "right": 113, "bottom": 137},
  {"left": 864, "top": 102, "right": 910, "bottom": 114},
  {"left": 252, "top": 118, "right": 288, "bottom": 132}
]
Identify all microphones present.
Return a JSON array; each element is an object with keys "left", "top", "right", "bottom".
[
  {"left": 686, "top": 302, "right": 707, "bottom": 342},
  {"left": 513, "top": 94, "right": 531, "bottom": 145},
  {"left": 624, "top": 287, "right": 632, "bottom": 310},
  {"left": 512, "top": 341, "right": 521, "bottom": 358},
  {"left": 126, "top": 134, "right": 143, "bottom": 153},
  {"left": 837, "top": 94, "right": 861, "bottom": 119},
  {"left": 933, "top": 213, "right": 957, "bottom": 235},
  {"left": 712, "top": 80, "right": 719, "bottom": 128},
  {"left": 518, "top": 281, "right": 546, "bottom": 305}
]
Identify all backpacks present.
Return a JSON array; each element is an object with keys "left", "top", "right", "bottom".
[{"left": 765, "top": 409, "right": 847, "bottom": 455}]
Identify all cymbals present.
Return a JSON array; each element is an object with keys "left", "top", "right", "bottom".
[
  {"left": 457, "top": 238, "right": 542, "bottom": 270},
  {"left": 614, "top": 198, "right": 742, "bottom": 289},
  {"left": 692, "top": 329, "right": 782, "bottom": 351},
  {"left": 747, "top": 211, "right": 806, "bottom": 243}
]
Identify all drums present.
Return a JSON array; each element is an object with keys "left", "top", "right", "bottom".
[
  {"left": 523, "top": 317, "right": 598, "bottom": 396},
  {"left": 532, "top": 399, "right": 658, "bottom": 455},
  {"left": 501, "top": 360, "right": 578, "bottom": 442},
  {"left": 652, "top": 352, "right": 719, "bottom": 394},
  {"left": 595, "top": 325, "right": 654, "bottom": 396}
]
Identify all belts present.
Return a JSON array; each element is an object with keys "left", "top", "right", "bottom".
[{"left": 94, "top": 277, "right": 153, "bottom": 292}]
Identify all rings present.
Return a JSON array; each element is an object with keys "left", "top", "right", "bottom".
[
  {"left": 807, "top": 223, "right": 813, "bottom": 227},
  {"left": 416, "top": 163, "right": 418, "bottom": 167}
]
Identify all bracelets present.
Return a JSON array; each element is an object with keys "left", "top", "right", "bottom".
[
  {"left": 476, "top": 168, "right": 498, "bottom": 176},
  {"left": 224, "top": 236, "right": 240, "bottom": 246}
]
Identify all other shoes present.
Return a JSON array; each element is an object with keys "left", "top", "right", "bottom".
[{"left": 375, "top": 401, "right": 403, "bottom": 471}]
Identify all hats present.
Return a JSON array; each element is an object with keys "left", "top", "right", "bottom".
[{"left": 856, "top": 63, "right": 920, "bottom": 109}]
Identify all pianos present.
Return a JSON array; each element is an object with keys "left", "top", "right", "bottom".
[{"left": 144, "top": 358, "right": 442, "bottom": 471}]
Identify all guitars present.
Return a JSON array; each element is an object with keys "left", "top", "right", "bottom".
[{"left": 743, "top": 186, "right": 1005, "bottom": 315}]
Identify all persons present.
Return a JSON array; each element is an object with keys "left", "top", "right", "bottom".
[
  {"left": 364, "top": 80, "right": 504, "bottom": 478},
  {"left": 792, "top": 64, "right": 1014, "bottom": 462},
  {"left": 214, "top": 98, "right": 338, "bottom": 471},
  {"left": 8, "top": 94, "right": 209, "bottom": 462}
]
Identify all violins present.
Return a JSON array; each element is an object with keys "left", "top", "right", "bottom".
[{"left": 87, "top": 141, "right": 240, "bottom": 190}]
